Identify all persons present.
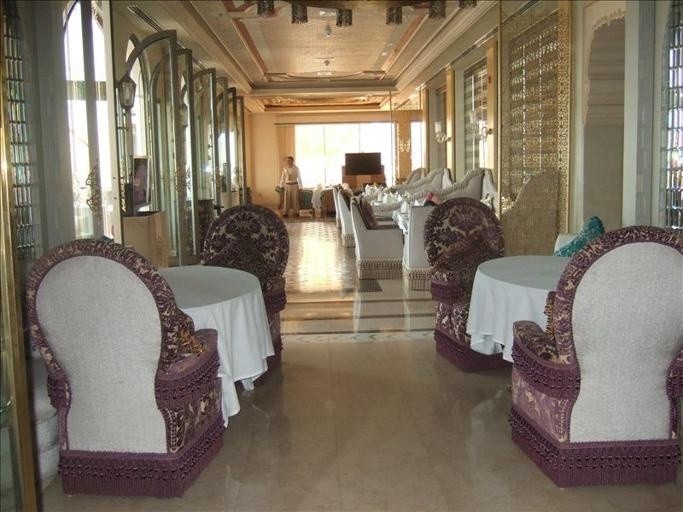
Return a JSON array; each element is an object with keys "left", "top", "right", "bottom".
[{"left": 280, "top": 156, "right": 303, "bottom": 217}]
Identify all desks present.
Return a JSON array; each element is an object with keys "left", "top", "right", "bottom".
[
  {"left": 146, "top": 262, "right": 279, "bottom": 430},
  {"left": 464, "top": 253, "right": 577, "bottom": 396}
]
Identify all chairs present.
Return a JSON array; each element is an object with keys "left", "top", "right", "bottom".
[
  {"left": 198, "top": 202, "right": 289, "bottom": 359},
  {"left": 419, "top": 193, "right": 520, "bottom": 377},
  {"left": 331, "top": 167, "right": 563, "bottom": 290},
  {"left": 25, "top": 237, "right": 226, "bottom": 498},
  {"left": 500, "top": 225, "right": 680, "bottom": 486}
]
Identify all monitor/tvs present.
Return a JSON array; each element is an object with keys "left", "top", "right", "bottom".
[{"left": 344, "top": 152, "right": 382, "bottom": 175}]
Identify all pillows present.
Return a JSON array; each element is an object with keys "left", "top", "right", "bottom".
[{"left": 551, "top": 214, "right": 605, "bottom": 257}]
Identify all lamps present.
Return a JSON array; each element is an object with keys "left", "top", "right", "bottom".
[{"left": 114, "top": 71, "right": 136, "bottom": 133}]
